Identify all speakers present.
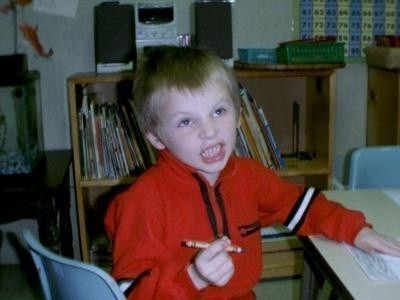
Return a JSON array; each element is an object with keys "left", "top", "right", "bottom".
[
  {"left": 195, "top": 2, "right": 233, "bottom": 69},
  {"left": 94, "top": 5, "right": 137, "bottom": 73}
]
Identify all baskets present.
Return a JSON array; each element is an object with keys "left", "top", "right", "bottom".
[
  {"left": 237, "top": 46, "right": 278, "bottom": 65},
  {"left": 275, "top": 41, "right": 346, "bottom": 65}
]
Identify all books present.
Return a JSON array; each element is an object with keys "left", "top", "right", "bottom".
[
  {"left": 77, "top": 88, "right": 159, "bottom": 182},
  {"left": 235, "top": 80, "right": 287, "bottom": 170}
]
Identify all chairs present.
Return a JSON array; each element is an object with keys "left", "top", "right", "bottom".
[
  {"left": 21, "top": 221, "right": 130, "bottom": 300},
  {"left": 347, "top": 143, "right": 400, "bottom": 192}
]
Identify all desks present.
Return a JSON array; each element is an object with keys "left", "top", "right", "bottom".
[
  {"left": 1, "top": 149, "right": 75, "bottom": 263},
  {"left": 297, "top": 187, "right": 400, "bottom": 300}
]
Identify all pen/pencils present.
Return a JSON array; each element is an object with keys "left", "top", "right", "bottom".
[{"left": 181, "top": 240, "right": 244, "bottom": 254}]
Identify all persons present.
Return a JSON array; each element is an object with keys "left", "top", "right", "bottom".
[{"left": 99, "top": 46, "right": 400, "bottom": 300}]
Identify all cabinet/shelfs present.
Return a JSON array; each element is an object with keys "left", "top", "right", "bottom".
[{"left": 65, "top": 70, "right": 336, "bottom": 281}]
